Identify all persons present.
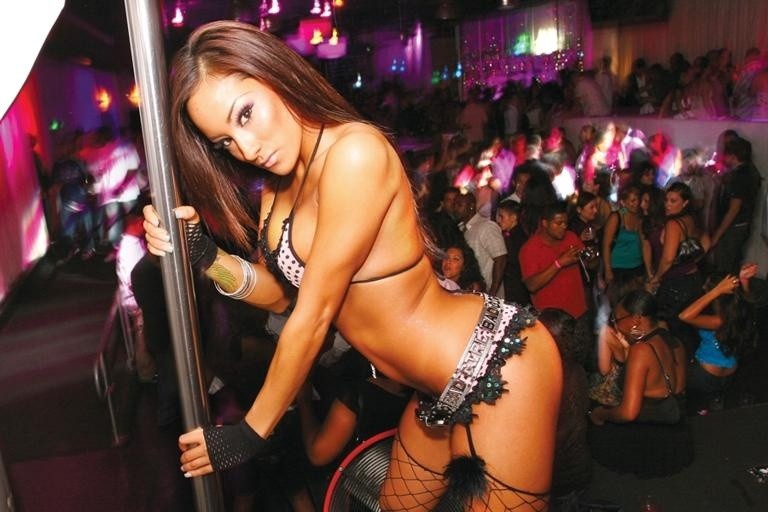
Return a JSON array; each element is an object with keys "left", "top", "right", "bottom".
[{"left": 29, "top": 19, "right": 768, "bottom": 512}]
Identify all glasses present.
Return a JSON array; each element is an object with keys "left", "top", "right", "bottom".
[{"left": 610, "top": 316, "right": 630, "bottom": 326}]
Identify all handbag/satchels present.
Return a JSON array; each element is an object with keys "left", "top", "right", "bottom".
[
  {"left": 630, "top": 428, "right": 694, "bottom": 483},
  {"left": 665, "top": 218, "right": 704, "bottom": 265}
]
